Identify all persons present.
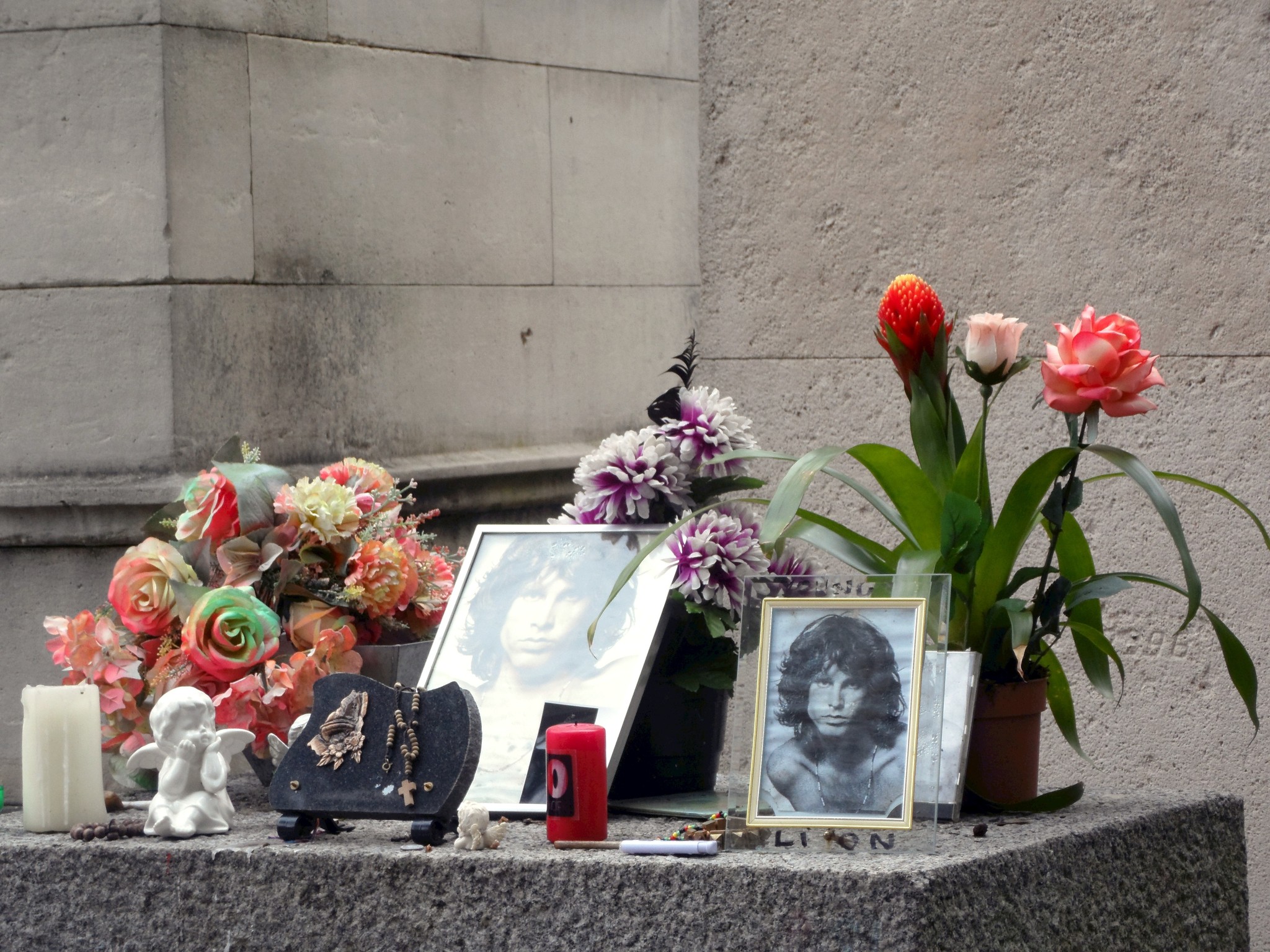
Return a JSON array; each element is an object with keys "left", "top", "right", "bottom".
[
  {"left": 759, "top": 610, "right": 903, "bottom": 819},
  {"left": 416, "top": 533, "right": 648, "bottom": 805}
]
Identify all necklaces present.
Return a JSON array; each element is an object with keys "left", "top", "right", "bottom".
[
  {"left": 464, "top": 678, "right": 583, "bottom": 774},
  {"left": 813, "top": 743, "right": 879, "bottom": 815}
]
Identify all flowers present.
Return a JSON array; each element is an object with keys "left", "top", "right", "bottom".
[
  {"left": 45, "top": 445, "right": 461, "bottom": 763},
  {"left": 586, "top": 262, "right": 1270, "bottom": 755},
  {"left": 543, "top": 336, "right": 822, "bottom": 663}
]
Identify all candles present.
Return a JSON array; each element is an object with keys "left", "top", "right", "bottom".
[
  {"left": 21, "top": 682, "right": 111, "bottom": 835},
  {"left": 545, "top": 713, "right": 607, "bottom": 842}
]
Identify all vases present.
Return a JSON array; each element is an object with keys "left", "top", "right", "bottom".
[
  {"left": 604, "top": 600, "right": 723, "bottom": 806},
  {"left": 940, "top": 660, "right": 1053, "bottom": 810},
  {"left": 239, "top": 639, "right": 435, "bottom": 789}
]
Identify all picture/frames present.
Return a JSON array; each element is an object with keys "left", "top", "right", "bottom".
[
  {"left": 414, "top": 522, "right": 692, "bottom": 816},
  {"left": 734, "top": 571, "right": 950, "bottom": 857}
]
